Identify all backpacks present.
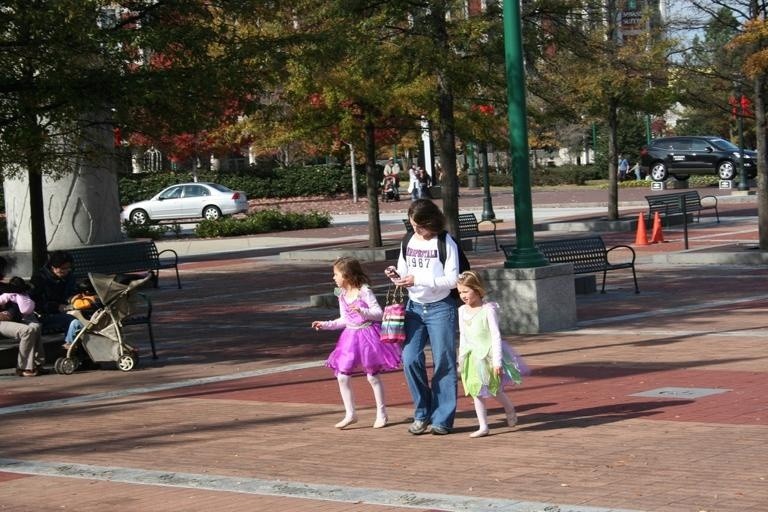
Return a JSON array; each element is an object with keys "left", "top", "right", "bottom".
[{"left": 402, "top": 229, "right": 470, "bottom": 299}]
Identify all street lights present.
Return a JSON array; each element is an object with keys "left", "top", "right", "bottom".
[
  {"left": 467, "top": 101, "right": 503, "bottom": 225},
  {"left": 580, "top": 115, "right": 591, "bottom": 168},
  {"left": 729, "top": 69, "right": 757, "bottom": 197}
]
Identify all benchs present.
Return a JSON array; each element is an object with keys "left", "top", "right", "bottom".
[
  {"left": 402, "top": 214, "right": 503, "bottom": 251},
  {"left": 499, "top": 236, "right": 640, "bottom": 295},
  {"left": 644, "top": 190, "right": 720, "bottom": 227},
  {"left": 48, "top": 238, "right": 185, "bottom": 291},
  {"left": 0, "top": 287, "right": 159, "bottom": 365}
]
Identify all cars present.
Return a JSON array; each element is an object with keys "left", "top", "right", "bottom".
[{"left": 120, "top": 180, "right": 249, "bottom": 228}]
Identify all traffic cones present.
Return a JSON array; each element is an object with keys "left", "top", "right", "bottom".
[
  {"left": 631, "top": 211, "right": 652, "bottom": 246},
  {"left": 649, "top": 212, "right": 670, "bottom": 243}
]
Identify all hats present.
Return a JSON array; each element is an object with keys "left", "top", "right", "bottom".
[{"left": 388, "top": 156, "right": 394, "bottom": 161}]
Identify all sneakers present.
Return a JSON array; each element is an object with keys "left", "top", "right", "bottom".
[
  {"left": 334, "top": 417, "right": 358, "bottom": 429},
  {"left": 429, "top": 424, "right": 453, "bottom": 436},
  {"left": 505, "top": 407, "right": 519, "bottom": 428},
  {"left": 469, "top": 429, "right": 490, "bottom": 438},
  {"left": 60, "top": 342, "right": 78, "bottom": 351},
  {"left": 408, "top": 416, "right": 432, "bottom": 435},
  {"left": 372, "top": 414, "right": 389, "bottom": 429},
  {"left": 15, "top": 365, "right": 51, "bottom": 377}
]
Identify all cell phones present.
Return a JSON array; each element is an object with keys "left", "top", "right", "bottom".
[{"left": 387, "top": 268, "right": 400, "bottom": 278}]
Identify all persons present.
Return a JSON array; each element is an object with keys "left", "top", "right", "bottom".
[
  {"left": 0, "top": 276, "right": 35, "bottom": 322},
  {"left": 453, "top": 269, "right": 519, "bottom": 439},
  {"left": 382, "top": 198, "right": 469, "bottom": 436},
  {"left": 0, "top": 255, "right": 50, "bottom": 377},
  {"left": 617, "top": 154, "right": 629, "bottom": 183},
  {"left": 59, "top": 279, "right": 102, "bottom": 351},
  {"left": 310, "top": 255, "right": 397, "bottom": 430},
  {"left": 407, "top": 164, "right": 431, "bottom": 202},
  {"left": 29, "top": 250, "right": 85, "bottom": 337},
  {"left": 628, "top": 161, "right": 641, "bottom": 180},
  {"left": 382, "top": 157, "right": 400, "bottom": 189},
  {"left": 385, "top": 181, "right": 393, "bottom": 192}
]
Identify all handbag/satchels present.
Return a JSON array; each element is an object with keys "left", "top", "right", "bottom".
[{"left": 380, "top": 302, "right": 406, "bottom": 344}]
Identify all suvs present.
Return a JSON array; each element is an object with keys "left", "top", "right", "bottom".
[{"left": 641, "top": 136, "right": 759, "bottom": 183}]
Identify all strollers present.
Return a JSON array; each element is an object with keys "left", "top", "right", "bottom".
[
  {"left": 53, "top": 268, "right": 157, "bottom": 372},
  {"left": 379, "top": 176, "right": 400, "bottom": 203}
]
